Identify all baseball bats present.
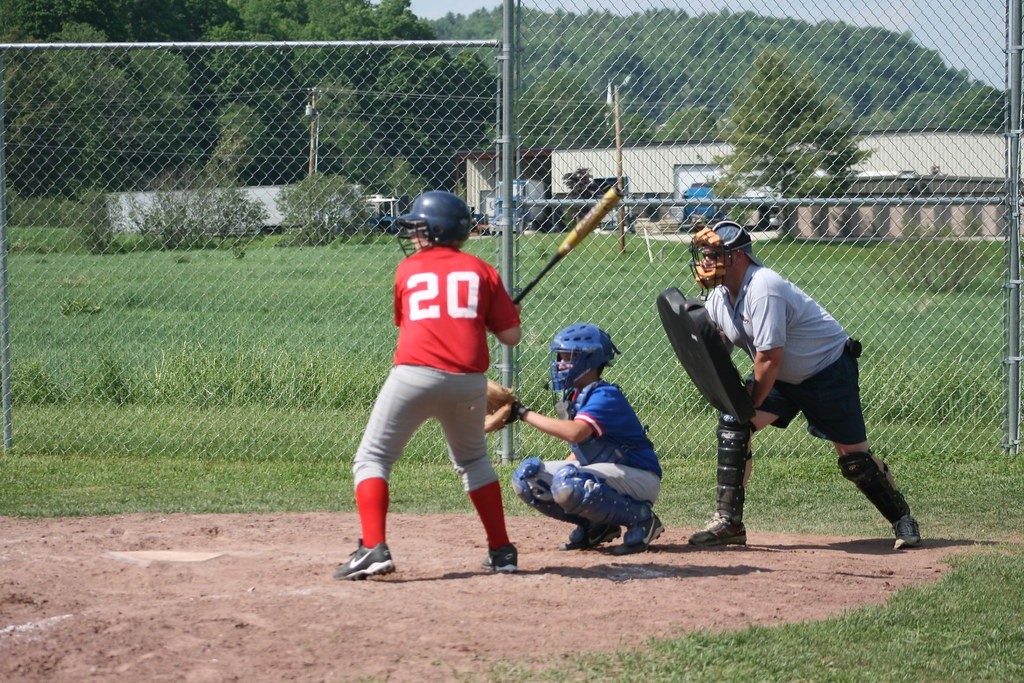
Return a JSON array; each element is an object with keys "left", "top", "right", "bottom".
[{"left": 512, "top": 185, "right": 624, "bottom": 306}]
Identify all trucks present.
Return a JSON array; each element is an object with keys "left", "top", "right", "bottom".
[
  {"left": 492, "top": 179, "right": 546, "bottom": 232},
  {"left": 683, "top": 184, "right": 779, "bottom": 230}
]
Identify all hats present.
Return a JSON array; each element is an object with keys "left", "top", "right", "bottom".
[{"left": 702, "top": 221, "right": 764, "bottom": 268}]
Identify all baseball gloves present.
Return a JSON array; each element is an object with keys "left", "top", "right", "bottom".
[{"left": 484, "top": 376, "right": 533, "bottom": 434}]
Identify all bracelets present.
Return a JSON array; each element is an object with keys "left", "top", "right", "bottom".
[{"left": 518, "top": 404, "right": 530, "bottom": 422}]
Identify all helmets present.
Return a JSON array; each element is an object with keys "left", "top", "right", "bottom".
[
  {"left": 550, "top": 324, "right": 614, "bottom": 392},
  {"left": 397, "top": 190, "right": 470, "bottom": 242}
]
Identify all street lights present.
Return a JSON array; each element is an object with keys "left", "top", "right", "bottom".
[{"left": 613, "top": 75, "right": 633, "bottom": 254}]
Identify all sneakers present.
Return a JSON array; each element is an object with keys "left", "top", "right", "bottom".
[
  {"left": 333, "top": 540, "right": 394, "bottom": 581},
  {"left": 559, "top": 520, "right": 623, "bottom": 551},
  {"left": 488, "top": 543, "right": 517, "bottom": 572},
  {"left": 613, "top": 510, "right": 665, "bottom": 554},
  {"left": 891, "top": 515, "right": 921, "bottom": 550},
  {"left": 688, "top": 512, "right": 746, "bottom": 548}
]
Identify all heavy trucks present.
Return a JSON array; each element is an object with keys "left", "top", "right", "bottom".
[{"left": 106, "top": 183, "right": 399, "bottom": 240}]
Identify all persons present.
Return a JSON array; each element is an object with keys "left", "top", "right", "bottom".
[
  {"left": 689, "top": 221, "right": 923, "bottom": 550},
  {"left": 484, "top": 324, "right": 665, "bottom": 556},
  {"left": 332, "top": 190, "right": 522, "bottom": 582}
]
